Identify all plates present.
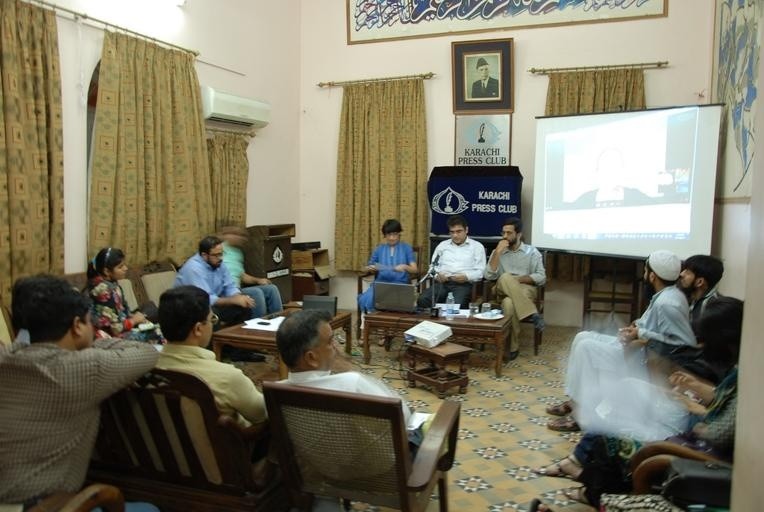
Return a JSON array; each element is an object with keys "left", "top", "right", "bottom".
[{"left": 473, "top": 312, "right": 504, "bottom": 321}]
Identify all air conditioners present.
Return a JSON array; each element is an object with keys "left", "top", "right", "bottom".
[{"left": 200, "top": 85, "right": 272, "bottom": 130}]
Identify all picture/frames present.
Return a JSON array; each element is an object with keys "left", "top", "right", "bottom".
[
  {"left": 346, "top": 0, "right": 669, "bottom": 46},
  {"left": 454, "top": 113, "right": 512, "bottom": 166},
  {"left": 450, "top": 37, "right": 515, "bottom": 114},
  {"left": 709, "top": 0, "right": 752, "bottom": 205}
]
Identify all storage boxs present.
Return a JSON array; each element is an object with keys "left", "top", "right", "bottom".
[{"left": 291, "top": 248, "right": 337, "bottom": 280}]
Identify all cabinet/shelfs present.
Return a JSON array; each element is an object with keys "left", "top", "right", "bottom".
[{"left": 292, "top": 269, "right": 330, "bottom": 302}]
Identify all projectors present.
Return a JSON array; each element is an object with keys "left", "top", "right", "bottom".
[{"left": 403, "top": 320, "right": 453, "bottom": 349}]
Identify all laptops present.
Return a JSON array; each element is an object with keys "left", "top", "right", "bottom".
[{"left": 373, "top": 282, "right": 417, "bottom": 312}]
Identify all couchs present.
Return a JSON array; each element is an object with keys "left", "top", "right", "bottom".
[{"left": 0, "top": 261, "right": 227, "bottom": 349}]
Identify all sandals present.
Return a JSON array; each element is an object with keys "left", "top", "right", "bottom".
[
  {"left": 548, "top": 413, "right": 580, "bottom": 431},
  {"left": 531, "top": 454, "right": 585, "bottom": 482},
  {"left": 562, "top": 487, "right": 589, "bottom": 505},
  {"left": 546, "top": 399, "right": 576, "bottom": 415},
  {"left": 529, "top": 498, "right": 552, "bottom": 512}
]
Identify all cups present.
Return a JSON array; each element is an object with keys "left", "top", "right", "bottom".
[
  {"left": 482, "top": 304, "right": 491, "bottom": 313},
  {"left": 469, "top": 303, "right": 478, "bottom": 317}
]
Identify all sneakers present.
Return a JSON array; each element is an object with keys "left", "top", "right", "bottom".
[{"left": 648, "top": 249, "right": 681, "bottom": 283}]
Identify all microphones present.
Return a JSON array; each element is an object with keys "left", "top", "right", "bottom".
[{"left": 431, "top": 250, "right": 443, "bottom": 266}]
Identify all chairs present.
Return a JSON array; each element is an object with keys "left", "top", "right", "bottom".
[
  {"left": 479, "top": 250, "right": 548, "bottom": 356},
  {"left": 356, "top": 246, "right": 423, "bottom": 340},
  {"left": 261, "top": 380, "right": 462, "bottom": 512},
  {"left": 421, "top": 247, "right": 488, "bottom": 348},
  {"left": 582, "top": 255, "right": 640, "bottom": 331},
  {"left": 88, "top": 366, "right": 296, "bottom": 512}
]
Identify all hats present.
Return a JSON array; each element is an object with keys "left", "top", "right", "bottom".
[{"left": 476, "top": 58, "right": 489, "bottom": 69}]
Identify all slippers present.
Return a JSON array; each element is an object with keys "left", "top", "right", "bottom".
[
  {"left": 359, "top": 336, "right": 364, "bottom": 347},
  {"left": 378, "top": 336, "right": 385, "bottom": 346}
]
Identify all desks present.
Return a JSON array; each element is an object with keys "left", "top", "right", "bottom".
[{"left": 212, "top": 307, "right": 353, "bottom": 381}]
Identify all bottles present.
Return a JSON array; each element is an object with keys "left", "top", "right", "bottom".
[{"left": 446, "top": 292, "right": 455, "bottom": 320}]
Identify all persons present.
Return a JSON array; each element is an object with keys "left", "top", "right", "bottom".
[
  {"left": 83, "top": 247, "right": 169, "bottom": 348},
  {"left": 358, "top": 219, "right": 418, "bottom": 311},
  {"left": 276, "top": 310, "right": 429, "bottom": 495},
  {"left": 0, "top": 277, "right": 159, "bottom": 510},
  {"left": 471, "top": 59, "right": 498, "bottom": 97},
  {"left": 486, "top": 218, "right": 546, "bottom": 358},
  {"left": 174, "top": 236, "right": 266, "bottom": 362},
  {"left": 156, "top": 284, "right": 268, "bottom": 487},
  {"left": 678, "top": 253, "right": 725, "bottom": 316},
  {"left": 532, "top": 295, "right": 743, "bottom": 512},
  {"left": 220, "top": 226, "right": 282, "bottom": 317},
  {"left": 417, "top": 216, "right": 487, "bottom": 311},
  {"left": 544, "top": 250, "right": 698, "bottom": 431}
]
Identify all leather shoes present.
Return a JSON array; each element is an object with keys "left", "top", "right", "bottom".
[
  {"left": 231, "top": 349, "right": 265, "bottom": 362},
  {"left": 531, "top": 313, "right": 545, "bottom": 332},
  {"left": 511, "top": 351, "right": 518, "bottom": 358}
]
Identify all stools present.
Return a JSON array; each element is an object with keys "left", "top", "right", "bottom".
[{"left": 407, "top": 341, "right": 474, "bottom": 399}]
